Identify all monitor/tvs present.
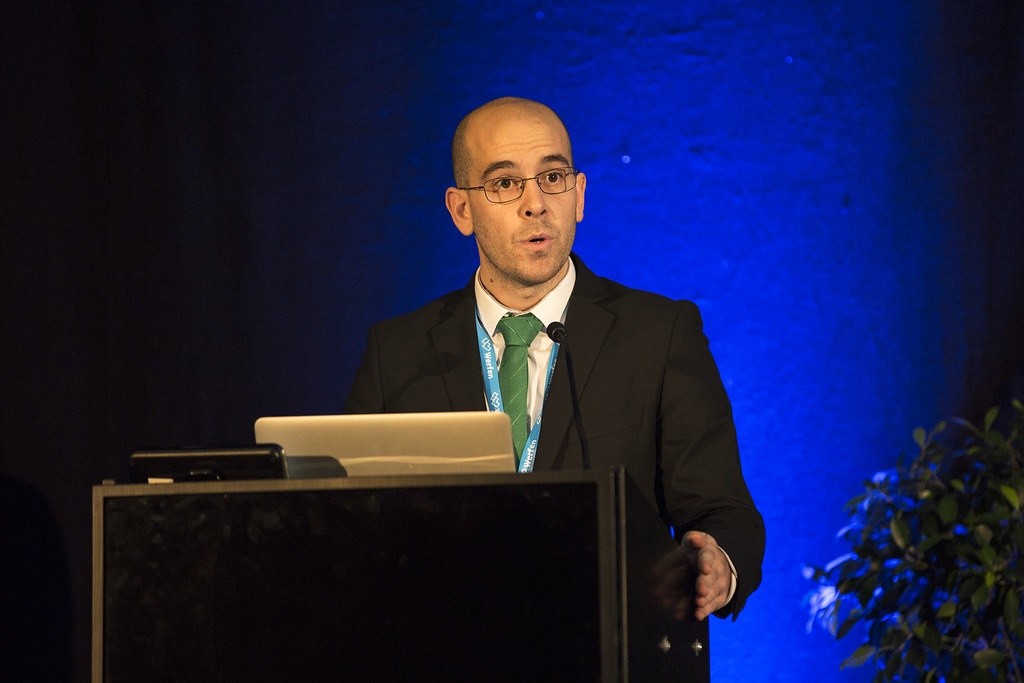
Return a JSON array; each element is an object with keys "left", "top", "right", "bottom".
[{"left": 130, "top": 441, "right": 288, "bottom": 483}]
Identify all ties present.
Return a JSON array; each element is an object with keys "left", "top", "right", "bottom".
[{"left": 497, "top": 313, "right": 544, "bottom": 470}]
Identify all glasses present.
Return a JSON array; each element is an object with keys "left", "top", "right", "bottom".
[{"left": 460, "top": 167, "right": 577, "bottom": 203}]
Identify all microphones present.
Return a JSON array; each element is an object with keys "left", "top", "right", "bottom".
[{"left": 546, "top": 321, "right": 590, "bottom": 469}]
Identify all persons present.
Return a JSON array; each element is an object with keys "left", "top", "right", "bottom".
[{"left": 350, "top": 96, "right": 766, "bottom": 623}]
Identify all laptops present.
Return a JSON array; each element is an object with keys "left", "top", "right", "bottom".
[{"left": 254, "top": 413, "right": 515, "bottom": 480}]
